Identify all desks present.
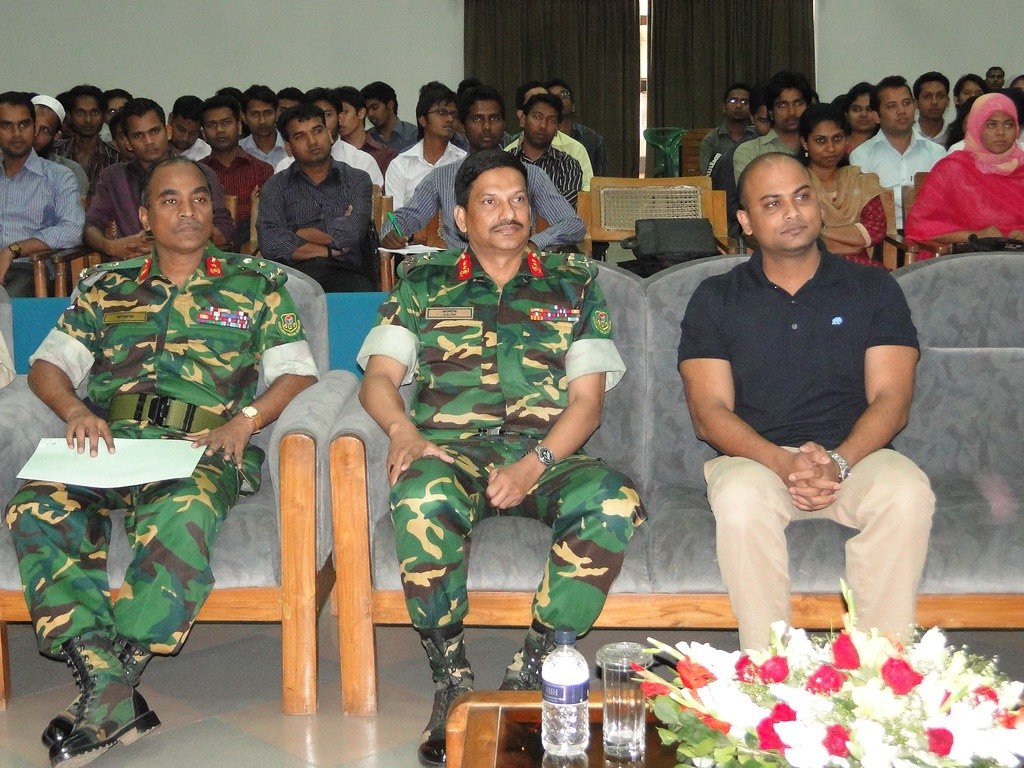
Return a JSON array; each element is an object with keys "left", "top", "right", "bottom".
[{"left": 446, "top": 689, "right": 697, "bottom": 768}]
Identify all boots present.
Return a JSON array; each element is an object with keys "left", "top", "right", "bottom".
[
  {"left": 41, "top": 628, "right": 164, "bottom": 768},
  {"left": 413, "top": 619, "right": 475, "bottom": 768},
  {"left": 496, "top": 619, "right": 556, "bottom": 731}
]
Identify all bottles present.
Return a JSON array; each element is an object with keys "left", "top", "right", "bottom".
[{"left": 540, "top": 626, "right": 591, "bottom": 758}]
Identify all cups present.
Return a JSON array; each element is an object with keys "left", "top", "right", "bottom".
[{"left": 595, "top": 640, "right": 654, "bottom": 760}]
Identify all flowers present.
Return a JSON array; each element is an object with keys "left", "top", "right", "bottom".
[{"left": 631, "top": 575, "right": 1024, "bottom": 768}]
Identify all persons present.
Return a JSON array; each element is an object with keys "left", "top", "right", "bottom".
[
  {"left": 0, "top": 79, "right": 594, "bottom": 292},
  {"left": 356, "top": 149, "right": 648, "bottom": 768},
  {"left": 675, "top": 152, "right": 937, "bottom": 660},
  {"left": 4, "top": 159, "right": 321, "bottom": 768},
  {"left": 700, "top": 67, "right": 1024, "bottom": 271}
]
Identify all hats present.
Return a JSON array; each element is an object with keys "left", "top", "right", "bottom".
[{"left": 30, "top": 94, "right": 66, "bottom": 125}]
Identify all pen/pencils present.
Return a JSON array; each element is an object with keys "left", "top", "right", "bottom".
[{"left": 387, "top": 212, "right": 407, "bottom": 248}]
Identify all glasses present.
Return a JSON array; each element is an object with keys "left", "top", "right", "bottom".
[
  {"left": 559, "top": 91, "right": 571, "bottom": 99},
  {"left": 728, "top": 99, "right": 749, "bottom": 106},
  {"left": 428, "top": 109, "right": 458, "bottom": 119}
]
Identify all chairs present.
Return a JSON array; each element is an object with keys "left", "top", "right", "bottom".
[{"left": 0, "top": 130, "right": 953, "bottom": 715}]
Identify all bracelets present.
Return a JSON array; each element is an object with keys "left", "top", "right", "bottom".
[{"left": 326, "top": 246, "right": 332, "bottom": 259}]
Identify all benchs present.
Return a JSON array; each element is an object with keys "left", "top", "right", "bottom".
[{"left": 325, "top": 252, "right": 1024, "bottom": 718}]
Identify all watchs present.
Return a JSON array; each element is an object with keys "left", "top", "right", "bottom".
[
  {"left": 238, "top": 406, "right": 262, "bottom": 436},
  {"left": 9, "top": 242, "right": 22, "bottom": 261},
  {"left": 532, "top": 445, "right": 555, "bottom": 468},
  {"left": 827, "top": 451, "right": 850, "bottom": 481}
]
